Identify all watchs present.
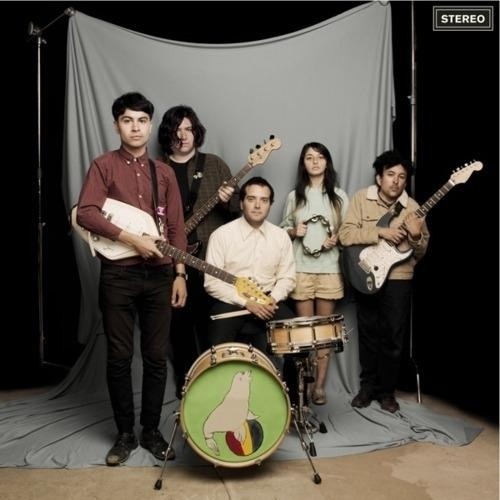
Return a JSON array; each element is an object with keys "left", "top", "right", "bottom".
[{"left": 173, "top": 272, "right": 188, "bottom": 281}]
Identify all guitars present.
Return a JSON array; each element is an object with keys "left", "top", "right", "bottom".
[
  {"left": 70, "top": 197, "right": 271, "bottom": 304},
  {"left": 183, "top": 135, "right": 282, "bottom": 253},
  {"left": 342, "top": 161, "right": 478, "bottom": 295}
]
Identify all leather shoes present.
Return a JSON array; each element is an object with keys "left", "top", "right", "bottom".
[{"left": 106, "top": 389, "right": 399, "bottom": 465}]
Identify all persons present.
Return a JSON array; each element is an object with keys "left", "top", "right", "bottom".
[
  {"left": 283, "top": 143, "right": 350, "bottom": 406},
  {"left": 77, "top": 94, "right": 189, "bottom": 466},
  {"left": 207, "top": 179, "right": 300, "bottom": 405},
  {"left": 159, "top": 107, "right": 243, "bottom": 397},
  {"left": 340, "top": 150, "right": 429, "bottom": 412}
]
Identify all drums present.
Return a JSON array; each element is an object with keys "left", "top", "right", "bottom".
[
  {"left": 265, "top": 315, "right": 346, "bottom": 356},
  {"left": 178, "top": 342, "right": 292, "bottom": 468}
]
